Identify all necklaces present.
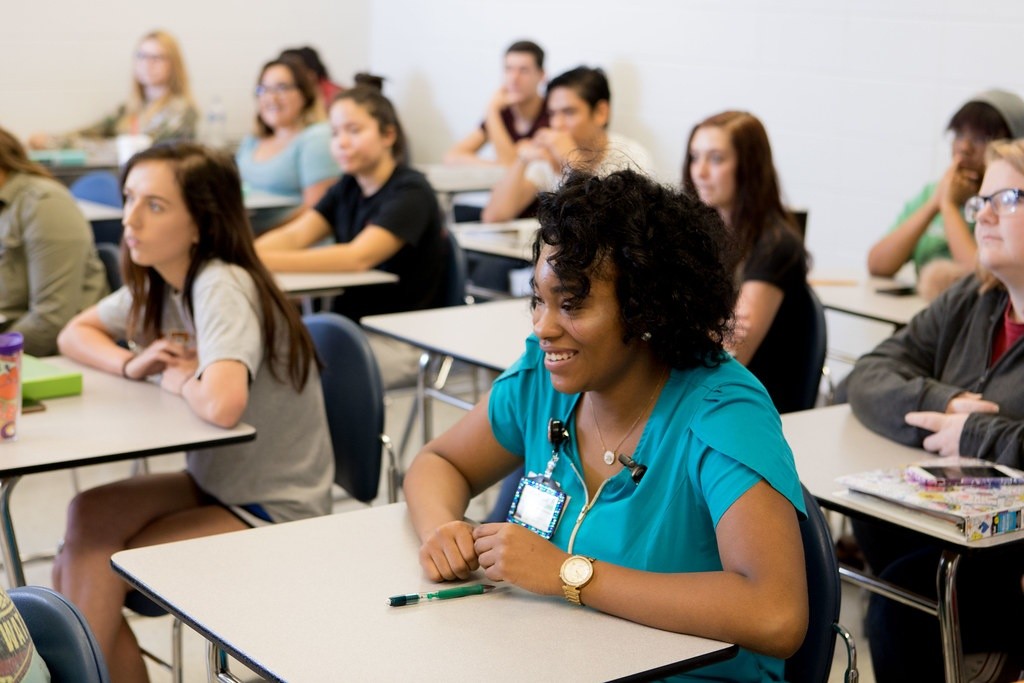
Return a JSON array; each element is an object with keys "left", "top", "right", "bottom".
[{"left": 588, "top": 365, "right": 669, "bottom": 465}]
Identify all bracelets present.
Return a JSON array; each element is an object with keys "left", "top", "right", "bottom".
[{"left": 122, "top": 356, "right": 146, "bottom": 381}]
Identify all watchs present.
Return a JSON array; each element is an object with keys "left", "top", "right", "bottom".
[{"left": 559, "top": 555, "right": 597, "bottom": 606}]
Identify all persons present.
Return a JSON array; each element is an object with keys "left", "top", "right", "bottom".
[
  {"left": 844, "top": 139, "right": 1024, "bottom": 683},
  {"left": 683, "top": 111, "right": 805, "bottom": 417},
  {"left": 53, "top": 136, "right": 332, "bottom": 683},
  {"left": 868, "top": 90, "right": 1024, "bottom": 306},
  {"left": 1, "top": 29, "right": 655, "bottom": 400},
  {"left": 402, "top": 164, "right": 808, "bottom": 683}
]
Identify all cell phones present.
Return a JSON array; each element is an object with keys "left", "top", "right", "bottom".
[{"left": 908, "top": 462, "right": 1024, "bottom": 487}]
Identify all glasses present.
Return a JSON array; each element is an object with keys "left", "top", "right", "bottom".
[
  {"left": 965, "top": 189, "right": 1023, "bottom": 224},
  {"left": 253, "top": 83, "right": 295, "bottom": 97},
  {"left": 132, "top": 50, "right": 169, "bottom": 64}
]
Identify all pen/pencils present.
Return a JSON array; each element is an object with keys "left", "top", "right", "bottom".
[{"left": 386, "top": 584, "right": 498, "bottom": 607}]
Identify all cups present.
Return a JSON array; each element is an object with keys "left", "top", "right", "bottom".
[{"left": 0, "top": 333, "right": 24, "bottom": 439}]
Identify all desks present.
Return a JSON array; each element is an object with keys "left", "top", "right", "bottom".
[
  {"left": 22, "top": 135, "right": 543, "bottom": 316},
  {"left": 1, "top": 352, "right": 256, "bottom": 591},
  {"left": 806, "top": 273, "right": 934, "bottom": 338},
  {"left": 359, "top": 293, "right": 536, "bottom": 487},
  {"left": 779, "top": 403, "right": 1024, "bottom": 683},
  {"left": 110, "top": 502, "right": 740, "bottom": 683}
]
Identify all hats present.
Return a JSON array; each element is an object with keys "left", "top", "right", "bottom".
[{"left": 942, "top": 91, "right": 1024, "bottom": 141}]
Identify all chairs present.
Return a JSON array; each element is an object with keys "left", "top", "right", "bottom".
[
  {"left": 394, "top": 230, "right": 482, "bottom": 462},
  {"left": 774, "top": 280, "right": 834, "bottom": 414},
  {"left": 784, "top": 479, "right": 860, "bottom": 683},
  {"left": 4, "top": 586, "right": 110, "bottom": 683},
  {"left": 121, "top": 312, "right": 399, "bottom": 682},
  {"left": 68, "top": 169, "right": 124, "bottom": 292}
]
